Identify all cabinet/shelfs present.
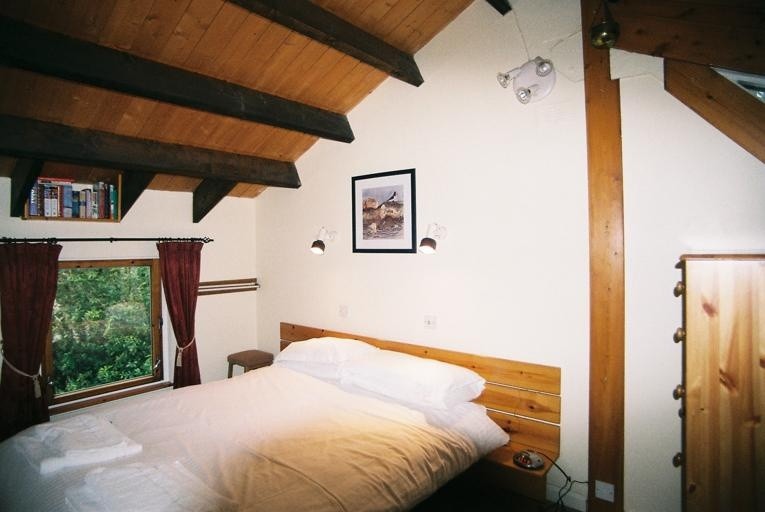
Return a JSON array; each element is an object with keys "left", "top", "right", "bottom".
[{"left": 669, "top": 253, "right": 764, "bottom": 511}]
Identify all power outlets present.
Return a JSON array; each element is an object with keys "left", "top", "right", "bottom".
[{"left": 591, "top": 478, "right": 615, "bottom": 503}]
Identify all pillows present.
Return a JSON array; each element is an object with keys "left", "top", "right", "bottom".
[{"left": 271, "top": 334, "right": 489, "bottom": 418}]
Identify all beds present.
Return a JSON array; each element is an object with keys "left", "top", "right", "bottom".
[{"left": 0, "top": 321, "right": 562, "bottom": 512}]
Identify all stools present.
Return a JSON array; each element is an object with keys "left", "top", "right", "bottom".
[{"left": 225, "top": 348, "right": 275, "bottom": 379}]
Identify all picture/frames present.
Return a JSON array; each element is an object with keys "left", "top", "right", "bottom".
[{"left": 349, "top": 168, "right": 416, "bottom": 254}]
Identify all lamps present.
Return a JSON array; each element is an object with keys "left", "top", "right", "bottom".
[
  {"left": 494, "top": 0, "right": 623, "bottom": 110},
  {"left": 307, "top": 223, "right": 341, "bottom": 255},
  {"left": 416, "top": 219, "right": 450, "bottom": 258}
]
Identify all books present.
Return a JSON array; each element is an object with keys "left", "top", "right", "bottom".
[{"left": 29, "top": 177, "right": 118, "bottom": 219}]
